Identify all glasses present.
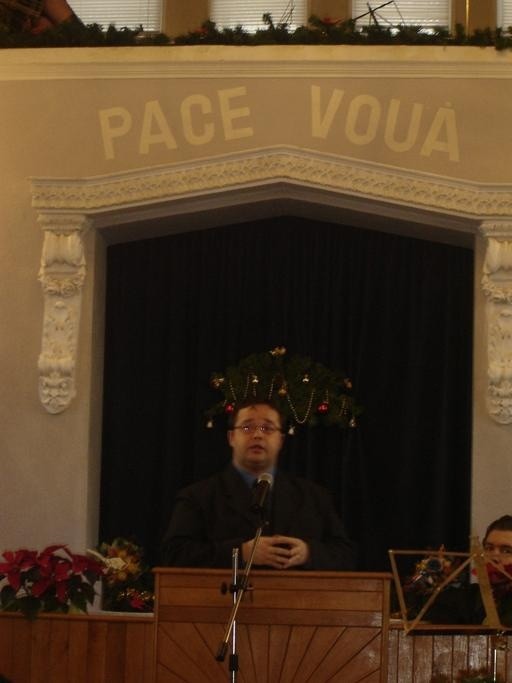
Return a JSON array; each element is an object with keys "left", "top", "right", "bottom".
[{"left": 234, "top": 423, "right": 282, "bottom": 434}]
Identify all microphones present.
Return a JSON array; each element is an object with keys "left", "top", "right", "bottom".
[{"left": 254, "top": 471, "right": 275, "bottom": 511}]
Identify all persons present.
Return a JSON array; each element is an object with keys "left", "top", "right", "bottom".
[
  {"left": 429, "top": 515, "right": 511, "bottom": 632},
  {"left": 159, "top": 401, "right": 359, "bottom": 571}
]
